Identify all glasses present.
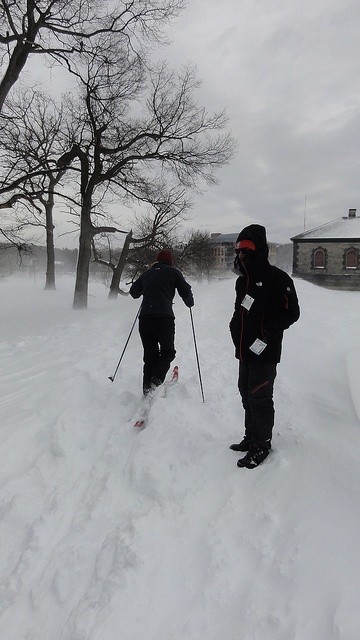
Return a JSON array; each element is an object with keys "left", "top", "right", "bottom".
[{"left": 235, "top": 249, "right": 250, "bottom": 255}]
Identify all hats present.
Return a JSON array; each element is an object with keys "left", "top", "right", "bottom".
[
  {"left": 156, "top": 250, "right": 172, "bottom": 266},
  {"left": 235, "top": 239, "right": 256, "bottom": 251}
]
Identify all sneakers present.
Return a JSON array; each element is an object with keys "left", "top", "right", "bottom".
[
  {"left": 230, "top": 436, "right": 255, "bottom": 451},
  {"left": 237, "top": 444, "right": 271, "bottom": 469}
]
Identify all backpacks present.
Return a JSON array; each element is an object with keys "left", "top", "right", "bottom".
[
  {"left": 271, "top": 265, "right": 300, "bottom": 331},
  {"left": 229, "top": 224, "right": 300, "bottom": 469}
]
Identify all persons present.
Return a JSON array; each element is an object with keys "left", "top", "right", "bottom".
[{"left": 128, "top": 250, "right": 195, "bottom": 397}]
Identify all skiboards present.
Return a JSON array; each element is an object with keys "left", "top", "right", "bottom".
[{"left": 126, "top": 366, "right": 178, "bottom": 429}]
